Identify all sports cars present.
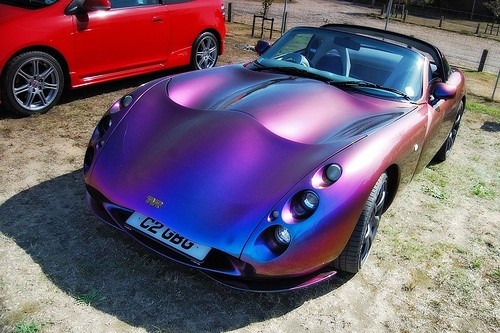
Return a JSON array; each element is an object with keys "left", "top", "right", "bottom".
[{"left": 84, "top": 23, "right": 467, "bottom": 293}]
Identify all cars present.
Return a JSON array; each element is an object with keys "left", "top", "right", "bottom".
[{"left": 0, "top": 1, "right": 227, "bottom": 116}]
